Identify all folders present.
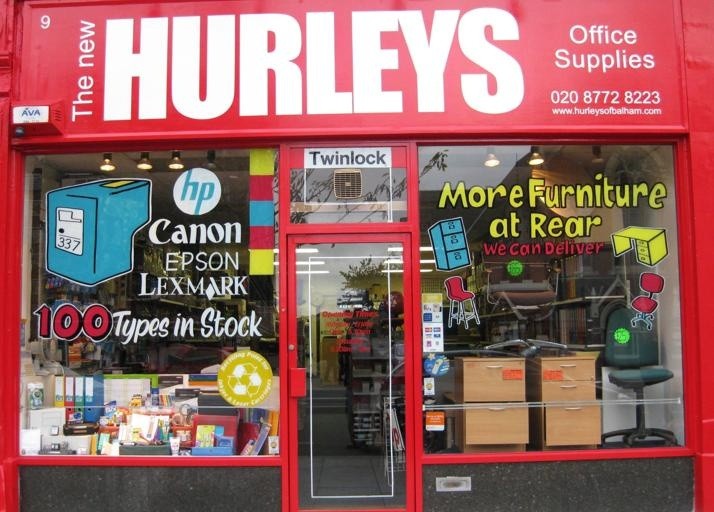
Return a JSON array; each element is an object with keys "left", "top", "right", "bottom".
[{"left": 54, "top": 374, "right": 103, "bottom": 425}]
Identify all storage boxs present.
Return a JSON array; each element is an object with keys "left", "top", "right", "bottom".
[{"left": 442, "top": 353, "right": 604, "bottom": 451}]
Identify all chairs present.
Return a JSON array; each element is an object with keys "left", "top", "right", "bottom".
[
  {"left": 483, "top": 249, "right": 568, "bottom": 355},
  {"left": 605, "top": 305, "right": 677, "bottom": 446}
]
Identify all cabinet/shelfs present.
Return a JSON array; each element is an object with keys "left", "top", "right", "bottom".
[{"left": 346, "top": 333, "right": 406, "bottom": 453}]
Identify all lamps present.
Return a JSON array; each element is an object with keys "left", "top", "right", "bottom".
[
  {"left": 529, "top": 146, "right": 544, "bottom": 166},
  {"left": 484, "top": 149, "right": 501, "bottom": 168},
  {"left": 203, "top": 151, "right": 217, "bottom": 168},
  {"left": 166, "top": 150, "right": 185, "bottom": 169},
  {"left": 100, "top": 152, "right": 117, "bottom": 171},
  {"left": 589, "top": 147, "right": 607, "bottom": 163},
  {"left": 136, "top": 152, "right": 152, "bottom": 170}
]
